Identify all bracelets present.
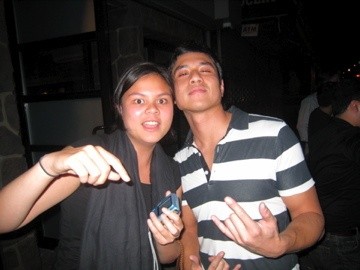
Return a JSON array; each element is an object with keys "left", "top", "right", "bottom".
[{"left": 38, "top": 153, "right": 62, "bottom": 178}]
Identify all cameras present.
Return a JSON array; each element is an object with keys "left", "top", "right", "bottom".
[{"left": 152, "top": 193, "right": 180, "bottom": 225}]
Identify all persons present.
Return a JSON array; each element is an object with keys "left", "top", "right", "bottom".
[
  {"left": 308, "top": 78, "right": 333, "bottom": 177},
  {"left": 0, "top": 61, "right": 185, "bottom": 270},
  {"left": 169, "top": 44, "right": 326, "bottom": 270},
  {"left": 305, "top": 72, "right": 360, "bottom": 270},
  {"left": 297, "top": 67, "right": 324, "bottom": 154}
]
